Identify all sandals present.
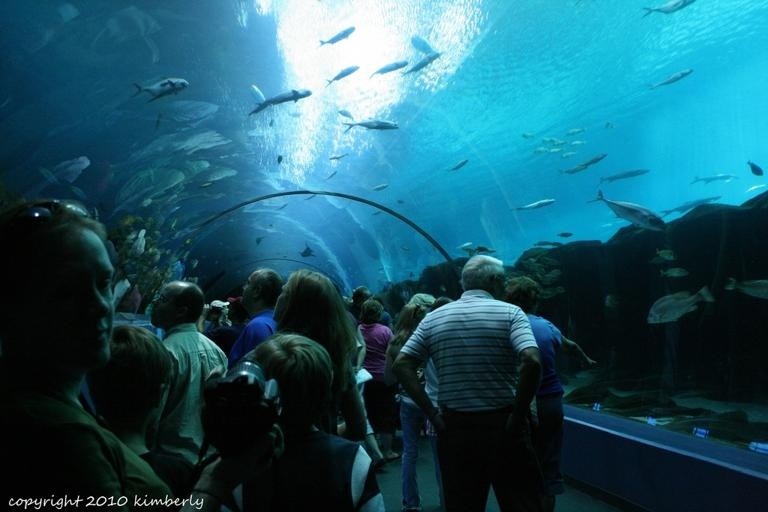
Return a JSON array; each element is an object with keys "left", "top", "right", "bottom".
[{"left": 383, "top": 451, "right": 402, "bottom": 461}]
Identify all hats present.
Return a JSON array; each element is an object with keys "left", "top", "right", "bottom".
[
  {"left": 211, "top": 300, "right": 230, "bottom": 308},
  {"left": 227, "top": 296, "right": 249, "bottom": 318}
]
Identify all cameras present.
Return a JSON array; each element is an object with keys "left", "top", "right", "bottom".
[{"left": 199, "top": 360, "right": 284, "bottom": 460}]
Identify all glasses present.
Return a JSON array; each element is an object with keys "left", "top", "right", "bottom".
[{"left": 9, "top": 199, "right": 93, "bottom": 229}]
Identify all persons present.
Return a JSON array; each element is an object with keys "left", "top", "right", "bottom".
[
  {"left": 199, "top": 257, "right": 456, "bottom": 512},
  {"left": 148, "top": 278, "right": 228, "bottom": 509},
  {"left": 2, "top": 197, "right": 287, "bottom": 511},
  {"left": 499, "top": 274, "right": 599, "bottom": 512},
  {"left": 392, "top": 254, "right": 543, "bottom": 512},
  {"left": 86, "top": 323, "right": 193, "bottom": 512}
]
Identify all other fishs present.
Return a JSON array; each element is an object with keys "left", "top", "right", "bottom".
[{"left": 0, "top": 0, "right": 768, "bottom": 324}]
